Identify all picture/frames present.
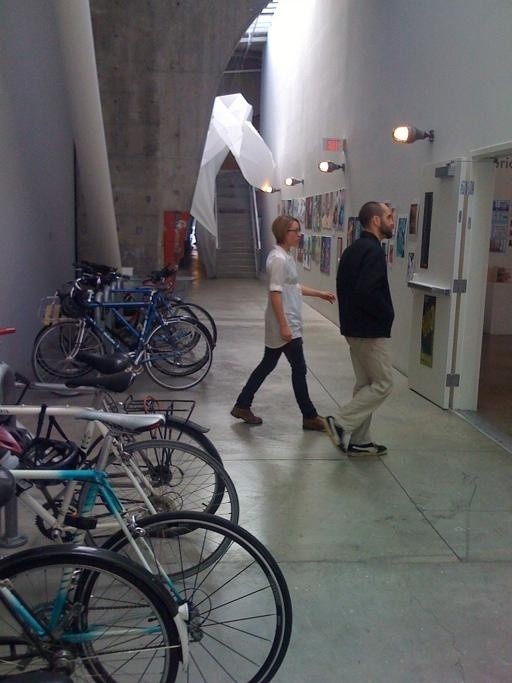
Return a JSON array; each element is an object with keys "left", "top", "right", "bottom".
[
  {"left": 320, "top": 235, "right": 332, "bottom": 274},
  {"left": 336, "top": 237, "right": 342, "bottom": 265},
  {"left": 395, "top": 214, "right": 408, "bottom": 258},
  {"left": 294, "top": 232, "right": 320, "bottom": 270},
  {"left": 406, "top": 248, "right": 415, "bottom": 286},
  {"left": 408, "top": 200, "right": 420, "bottom": 237},
  {"left": 277, "top": 187, "right": 346, "bottom": 231},
  {"left": 380, "top": 239, "right": 394, "bottom": 265},
  {"left": 346, "top": 216, "right": 361, "bottom": 247}
]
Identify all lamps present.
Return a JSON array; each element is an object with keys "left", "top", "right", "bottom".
[
  {"left": 266, "top": 185, "right": 279, "bottom": 193},
  {"left": 318, "top": 160, "right": 344, "bottom": 172},
  {"left": 392, "top": 125, "right": 434, "bottom": 143},
  {"left": 285, "top": 177, "right": 303, "bottom": 185}
]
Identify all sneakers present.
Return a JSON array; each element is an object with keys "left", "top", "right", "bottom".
[
  {"left": 348, "top": 441, "right": 387, "bottom": 456},
  {"left": 324, "top": 416, "right": 346, "bottom": 453}
]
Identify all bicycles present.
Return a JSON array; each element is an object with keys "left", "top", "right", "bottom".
[
  {"left": 0, "top": 370, "right": 240, "bottom": 581},
  {"left": 0, "top": 411, "right": 293, "bottom": 682},
  {"left": 31, "top": 258, "right": 217, "bottom": 397},
  {"left": 0, "top": 543, "right": 189, "bottom": 681},
  {"left": 0, "top": 327, "right": 226, "bottom": 539}
]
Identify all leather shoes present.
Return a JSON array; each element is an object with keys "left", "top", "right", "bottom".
[
  {"left": 302, "top": 414, "right": 325, "bottom": 431},
  {"left": 231, "top": 405, "right": 262, "bottom": 424}
]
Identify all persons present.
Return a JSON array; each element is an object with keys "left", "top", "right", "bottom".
[
  {"left": 323, "top": 201, "right": 396, "bottom": 454},
  {"left": 230, "top": 215, "right": 335, "bottom": 432}
]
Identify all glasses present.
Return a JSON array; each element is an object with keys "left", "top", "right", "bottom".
[{"left": 289, "top": 228, "right": 298, "bottom": 234}]
